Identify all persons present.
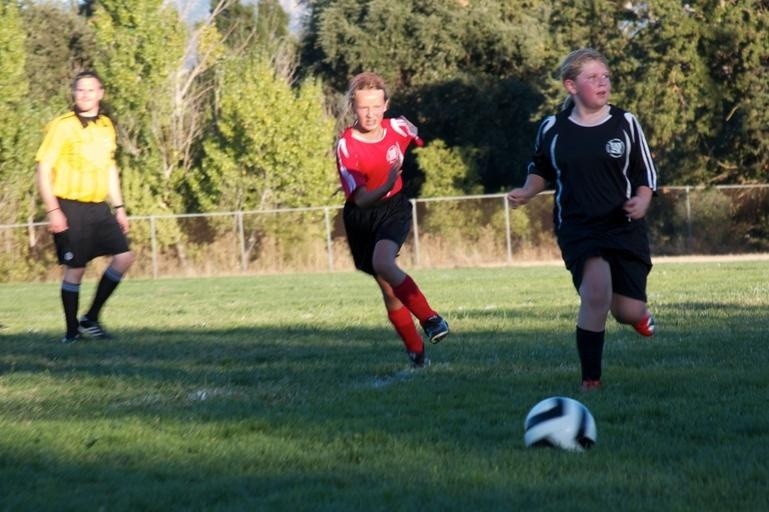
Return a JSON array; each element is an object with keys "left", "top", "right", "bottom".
[
  {"left": 32, "top": 71, "right": 135, "bottom": 342},
  {"left": 505, "top": 49, "right": 658, "bottom": 395},
  {"left": 331, "top": 73, "right": 451, "bottom": 371}
]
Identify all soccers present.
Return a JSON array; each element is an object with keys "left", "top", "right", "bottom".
[{"left": 524, "top": 397, "right": 597, "bottom": 453}]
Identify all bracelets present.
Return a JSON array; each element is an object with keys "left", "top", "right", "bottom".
[
  {"left": 112, "top": 204, "right": 123, "bottom": 209},
  {"left": 43, "top": 207, "right": 63, "bottom": 214}
]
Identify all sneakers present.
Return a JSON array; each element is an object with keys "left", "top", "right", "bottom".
[
  {"left": 405, "top": 357, "right": 431, "bottom": 372},
  {"left": 581, "top": 378, "right": 601, "bottom": 391},
  {"left": 632, "top": 311, "right": 655, "bottom": 336},
  {"left": 423, "top": 315, "right": 448, "bottom": 344},
  {"left": 61, "top": 315, "right": 106, "bottom": 344}
]
